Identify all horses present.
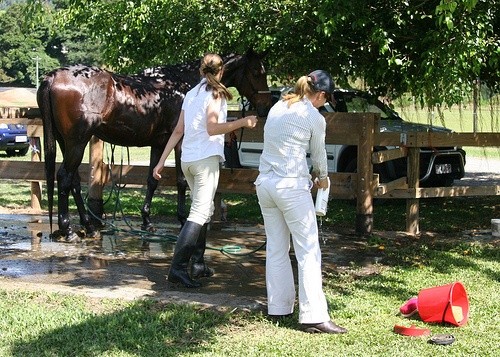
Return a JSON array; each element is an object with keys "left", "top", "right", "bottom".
[{"left": 36, "top": 45, "right": 276, "bottom": 244}]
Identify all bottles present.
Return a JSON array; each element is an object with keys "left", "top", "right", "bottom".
[{"left": 315, "top": 176, "right": 331, "bottom": 216}]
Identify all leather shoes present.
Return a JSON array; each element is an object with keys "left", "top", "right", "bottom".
[{"left": 301, "top": 321, "right": 348, "bottom": 334}]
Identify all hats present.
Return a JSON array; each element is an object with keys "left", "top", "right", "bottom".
[{"left": 307, "top": 70, "right": 335, "bottom": 102}]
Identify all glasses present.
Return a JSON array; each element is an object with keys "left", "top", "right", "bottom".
[{"left": 325, "top": 94, "right": 331, "bottom": 103}]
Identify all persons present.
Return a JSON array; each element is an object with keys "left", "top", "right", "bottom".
[
  {"left": 153, "top": 54, "right": 258, "bottom": 288},
  {"left": 254, "top": 70, "right": 348, "bottom": 334}
]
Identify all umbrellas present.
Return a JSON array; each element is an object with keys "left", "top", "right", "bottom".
[{"left": 0, "top": 88, "right": 39, "bottom": 108}]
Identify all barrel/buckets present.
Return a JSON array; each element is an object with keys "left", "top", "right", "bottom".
[{"left": 418, "top": 282, "right": 469, "bottom": 326}]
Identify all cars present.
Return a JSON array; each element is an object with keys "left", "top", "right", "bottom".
[
  {"left": 0, "top": 123, "right": 30, "bottom": 158},
  {"left": 221, "top": 84, "right": 466, "bottom": 206}
]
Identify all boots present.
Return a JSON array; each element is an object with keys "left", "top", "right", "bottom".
[{"left": 167, "top": 220, "right": 216, "bottom": 288}]
